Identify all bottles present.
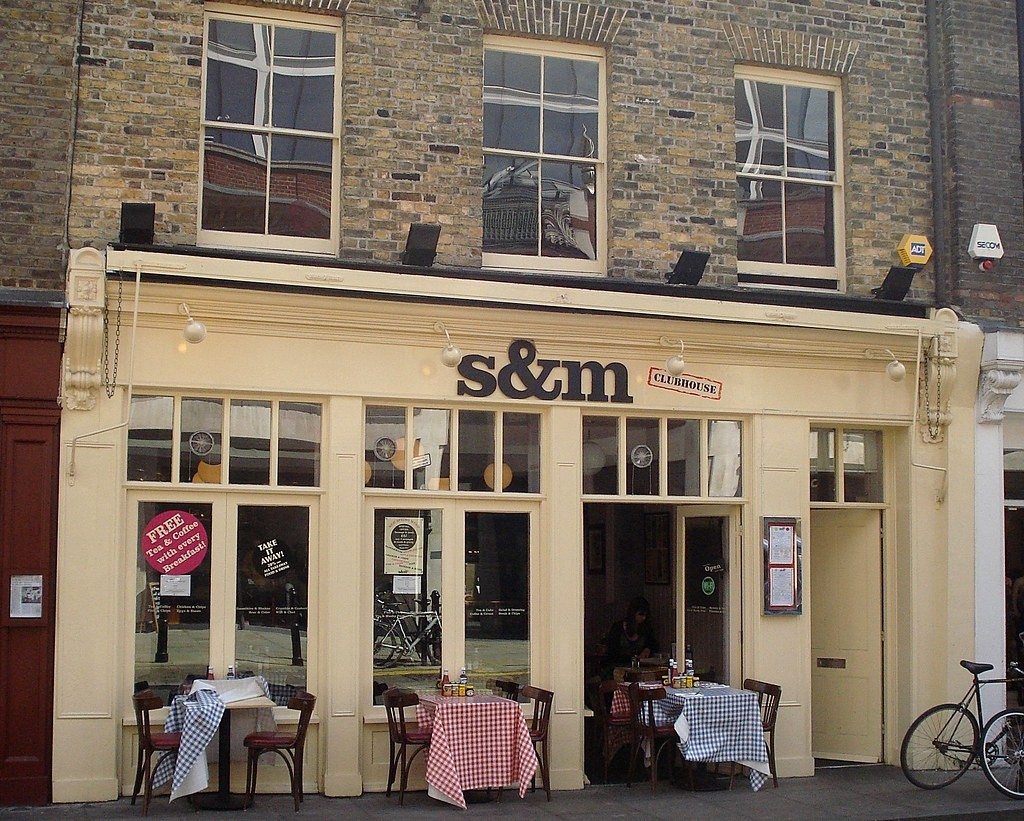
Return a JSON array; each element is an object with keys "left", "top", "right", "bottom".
[
  {"left": 442, "top": 669, "right": 449, "bottom": 696},
  {"left": 227, "top": 666, "right": 234, "bottom": 680},
  {"left": 669, "top": 659, "right": 693, "bottom": 687},
  {"left": 460, "top": 667, "right": 468, "bottom": 684},
  {"left": 208, "top": 667, "right": 214, "bottom": 680},
  {"left": 631, "top": 655, "right": 640, "bottom": 669}
]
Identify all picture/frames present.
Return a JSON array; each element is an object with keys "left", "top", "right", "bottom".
[{"left": 586, "top": 523, "right": 606, "bottom": 574}]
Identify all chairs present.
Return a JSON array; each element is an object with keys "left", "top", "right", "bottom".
[
  {"left": 383, "top": 688, "right": 432, "bottom": 806},
  {"left": 131, "top": 688, "right": 183, "bottom": 816},
  {"left": 373, "top": 681, "right": 388, "bottom": 706},
  {"left": 496, "top": 680, "right": 521, "bottom": 702},
  {"left": 243, "top": 690, "right": 317, "bottom": 813},
  {"left": 597, "top": 678, "right": 782, "bottom": 795},
  {"left": 486, "top": 684, "right": 554, "bottom": 802}
]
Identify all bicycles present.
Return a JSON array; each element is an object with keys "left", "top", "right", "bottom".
[
  {"left": 372, "top": 589, "right": 442, "bottom": 669},
  {"left": 899, "top": 632, "right": 1024, "bottom": 801}
]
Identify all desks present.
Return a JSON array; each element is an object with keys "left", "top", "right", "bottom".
[
  {"left": 413, "top": 690, "right": 540, "bottom": 809},
  {"left": 603, "top": 682, "right": 771, "bottom": 793},
  {"left": 152, "top": 676, "right": 278, "bottom": 813}
]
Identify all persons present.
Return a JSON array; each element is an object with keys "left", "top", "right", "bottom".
[{"left": 607, "top": 595, "right": 657, "bottom": 679}]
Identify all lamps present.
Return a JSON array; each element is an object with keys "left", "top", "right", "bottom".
[
  {"left": 401, "top": 222, "right": 441, "bottom": 267},
  {"left": 118, "top": 202, "right": 155, "bottom": 245},
  {"left": 870, "top": 265, "right": 916, "bottom": 301},
  {"left": 865, "top": 348, "right": 906, "bottom": 382},
  {"left": 583, "top": 429, "right": 605, "bottom": 476},
  {"left": 177, "top": 302, "right": 207, "bottom": 344},
  {"left": 660, "top": 336, "right": 685, "bottom": 377},
  {"left": 666, "top": 249, "right": 712, "bottom": 285},
  {"left": 434, "top": 322, "right": 462, "bottom": 367}
]
